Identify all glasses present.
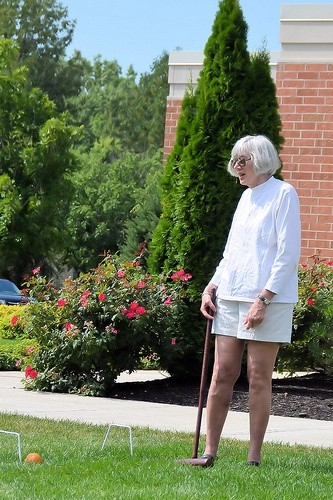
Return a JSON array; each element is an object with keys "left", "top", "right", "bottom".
[{"left": 230, "top": 158, "right": 251, "bottom": 168}]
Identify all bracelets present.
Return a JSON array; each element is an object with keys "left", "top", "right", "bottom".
[{"left": 202, "top": 291, "right": 214, "bottom": 297}]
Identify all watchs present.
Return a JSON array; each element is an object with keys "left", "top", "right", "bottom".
[{"left": 256, "top": 295, "right": 271, "bottom": 307}]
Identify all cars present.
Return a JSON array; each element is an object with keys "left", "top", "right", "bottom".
[{"left": 0, "top": 279, "right": 38, "bottom": 305}]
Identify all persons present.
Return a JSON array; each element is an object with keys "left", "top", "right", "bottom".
[{"left": 197, "top": 135, "right": 302, "bottom": 469}]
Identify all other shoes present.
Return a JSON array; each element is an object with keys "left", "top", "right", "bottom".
[
  {"left": 246, "top": 459, "right": 261, "bottom": 469},
  {"left": 199, "top": 453, "right": 219, "bottom": 469}
]
